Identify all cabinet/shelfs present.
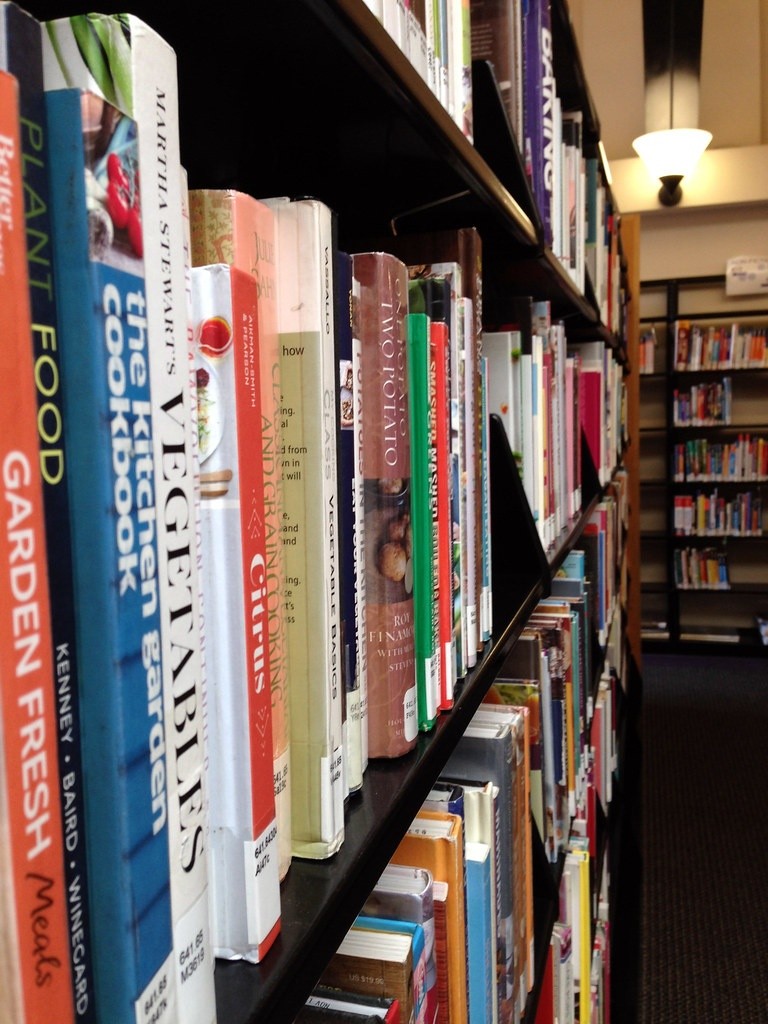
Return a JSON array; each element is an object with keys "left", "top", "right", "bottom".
[
  {"left": 0, "top": 0, "right": 632, "bottom": 1024},
  {"left": 639, "top": 273, "right": 768, "bottom": 661}
]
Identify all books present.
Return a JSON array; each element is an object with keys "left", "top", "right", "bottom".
[
  {"left": 639, "top": 328, "right": 655, "bottom": 374},
  {"left": 673, "top": 546, "right": 730, "bottom": 589},
  {"left": 673, "top": 487, "right": 763, "bottom": 537},
  {"left": 0, "top": 0, "right": 632, "bottom": 1024},
  {"left": 673, "top": 434, "right": 768, "bottom": 483},
  {"left": 673, "top": 377, "right": 732, "bottom": 427},
  {"left": 674, "top": 320, "right": 768, "bottom": 371}
]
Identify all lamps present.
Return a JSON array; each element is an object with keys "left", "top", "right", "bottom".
[{"left": 630, "top": 0, "right": 714, "bottom": 208}]
